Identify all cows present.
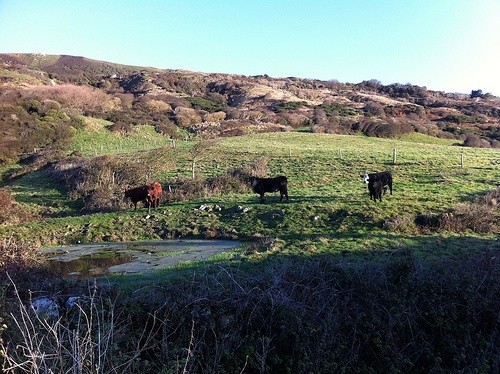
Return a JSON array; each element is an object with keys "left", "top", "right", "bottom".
[
  {"left": 359, "top": 170, "right": 393, "bottom": 203},
  {"left": 123, "top": 184, "right": 160, "bottom": 211},
  {"left": 142, "top": 181, "right": 162, "bottom": 215},
  {"left": 247, "top": 175, "right": 290, "bottom": 205}
]
